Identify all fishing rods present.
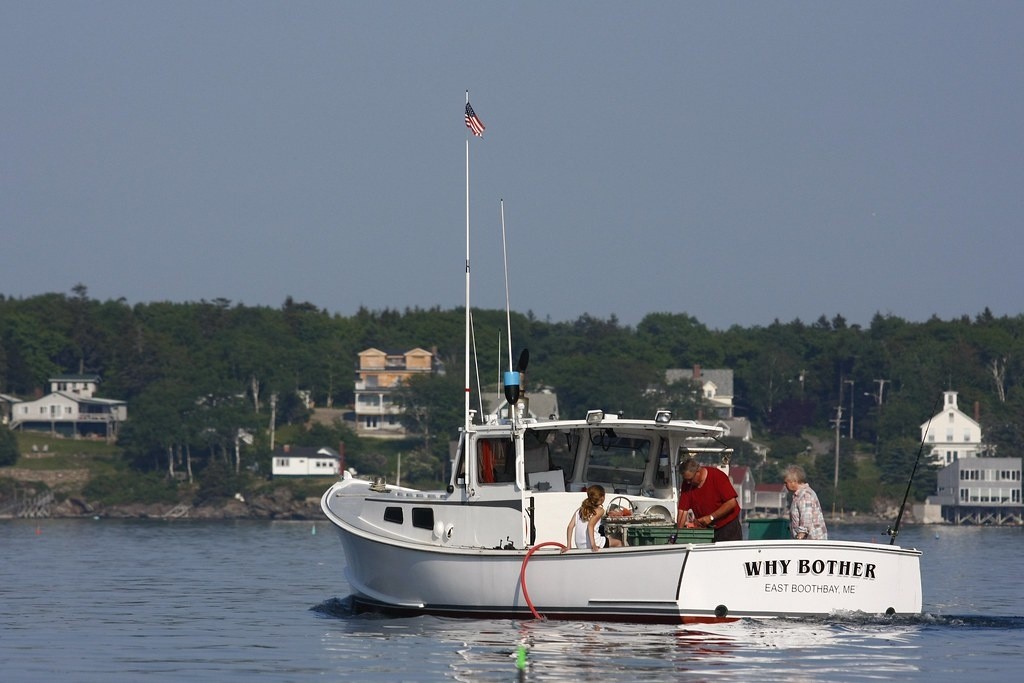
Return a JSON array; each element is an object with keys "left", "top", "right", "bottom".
[{"left": 880, "top": 380, "right": 948, "bottom": 545}]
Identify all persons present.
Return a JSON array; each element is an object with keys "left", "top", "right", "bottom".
[
  {"left": 783, "top": 465, "right": 827, "bottom": 540},
  {"left": 676, "top": 459, "right": 743, "bottom": 543},
  {"left": 560, "top": 484, "right": 622, "bottom": 553}
]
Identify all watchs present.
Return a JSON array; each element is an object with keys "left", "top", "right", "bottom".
[{"left": 710, "top": 515, "right": 714, "bottom": 520}]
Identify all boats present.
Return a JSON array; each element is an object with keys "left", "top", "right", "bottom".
[{"left": 320, "top": 82, "right": 925, "bottom": 622}]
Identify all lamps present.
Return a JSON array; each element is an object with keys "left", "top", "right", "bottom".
[
  {"left": 586, "top": 409, "right": 603, "bottom": 423},
  {"left": 655, "top": 411, "right": 672, "bottom": 426}
]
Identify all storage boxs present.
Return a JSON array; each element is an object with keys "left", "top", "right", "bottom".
[
  {"left": 627, "top": 526, "right": 714, "bottom": 546},
  {"left": 746, "top": 518, "right": 791, "bottom": 540}
]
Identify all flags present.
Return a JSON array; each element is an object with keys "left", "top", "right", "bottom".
[{"left": 465, "top": 102, "right": 485, "bottom": 137}]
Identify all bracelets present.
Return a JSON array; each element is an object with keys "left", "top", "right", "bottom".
[{"left": 796, "top": 536, "right": 802, "bottom": 539}]
